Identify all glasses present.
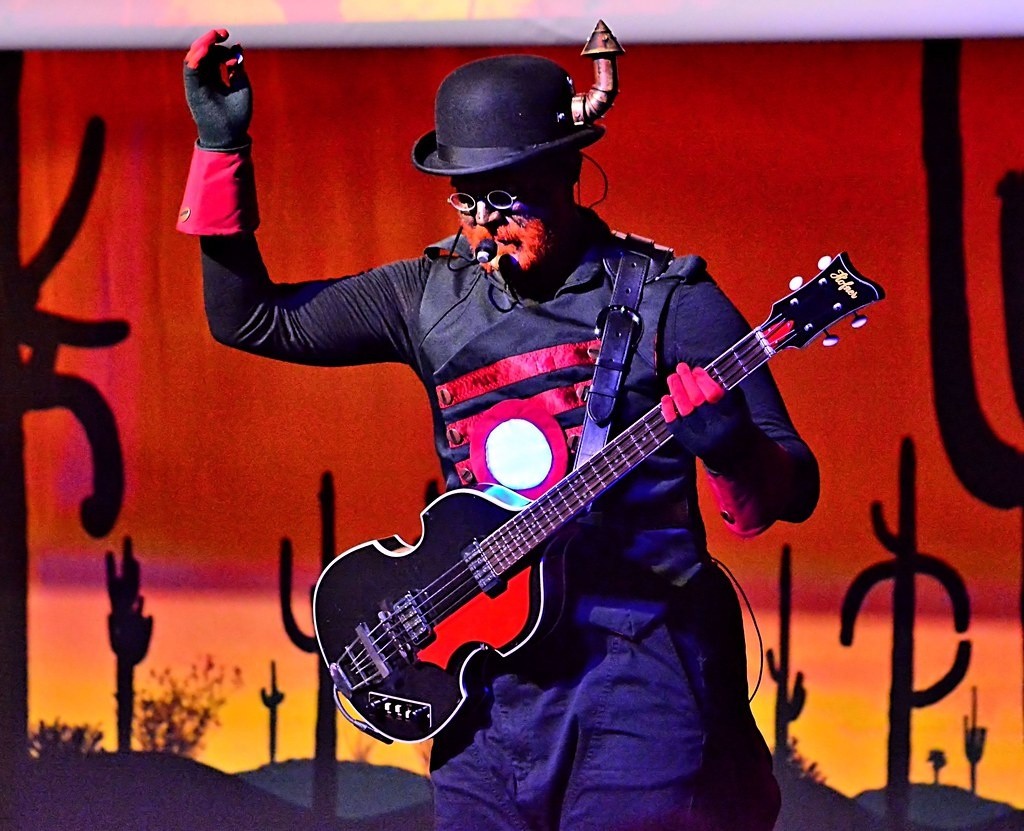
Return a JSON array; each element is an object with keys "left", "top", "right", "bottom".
[{"left": 446, "top": 166, "right": 555, "bottom": 213}]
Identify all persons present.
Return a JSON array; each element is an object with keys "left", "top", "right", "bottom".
[{"left": 176, "top": 20, "right": 822, "bottom": 831}]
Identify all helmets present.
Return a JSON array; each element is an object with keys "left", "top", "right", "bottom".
[{"left": 413, "top": 19, "right": 624, "bottom": 176}]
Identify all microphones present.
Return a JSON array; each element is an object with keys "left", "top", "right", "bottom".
[{"left": 447, "top": 225, "right": 498, "bottom": 273}]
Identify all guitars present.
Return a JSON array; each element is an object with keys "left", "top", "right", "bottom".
[{"left": 313, "top": 251, "right": 888, "bottom": 746}]
